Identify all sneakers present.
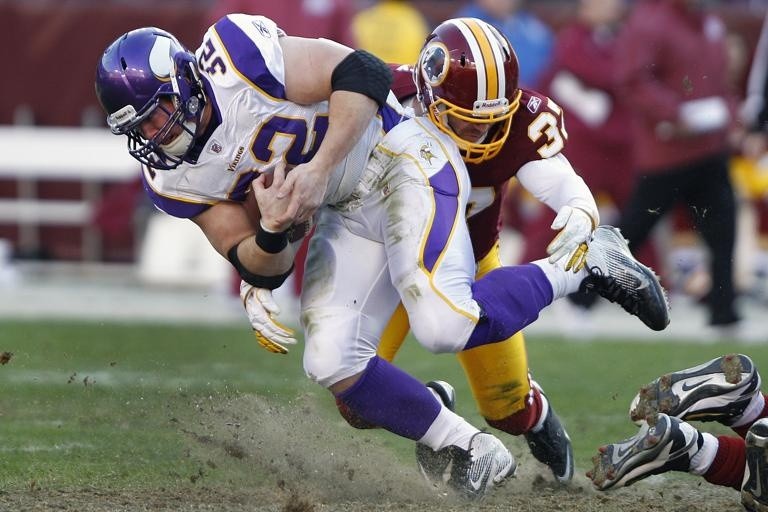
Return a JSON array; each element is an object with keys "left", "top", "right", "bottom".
[
  {"left": 415, "top": 380, "right": 516, "bottom": 500},
  {"left": 524, "top": 379, "right": 574, "bottom": 485},
  {"left": 586, "top": 353, "right": 768, "bottom": 510},
  {"left": 576, "top": 225, "right": 670, "bottom": 331}
]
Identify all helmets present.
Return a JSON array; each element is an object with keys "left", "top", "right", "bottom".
[
  {"left": 412, "top": 16, "right": 522, "bottom": 124},
  {"left": 95, "top": 27, "right": 206, "bottom": 135}
]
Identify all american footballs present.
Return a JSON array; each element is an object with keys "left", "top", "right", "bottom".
[{"left": 245, "top": 165, "right": 296, "bottom": 234}]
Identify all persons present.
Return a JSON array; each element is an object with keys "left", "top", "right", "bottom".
[
  {"left": 92, "top": 13, "right": 672, "bottom": 503},
  {"left": 585, "top": 350, "right": 768, "bottom": 496},
  {"left": 88, "top": 1, "right": 766, "bottom": 343},
  {"left": 239, "top": 13, "right": 602, "bottom": 496}
]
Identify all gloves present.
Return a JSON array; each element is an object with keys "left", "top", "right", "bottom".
[
  {"left": 238, "top": 280, "right": 298, "bottom": 356},
  {"left": 546, "top": 205, "right": 595, "bottom": 274}
]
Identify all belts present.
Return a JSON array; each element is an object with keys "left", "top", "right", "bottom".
[{"left": 334, "top": 156, "right": 384, "bottom": 211}]
